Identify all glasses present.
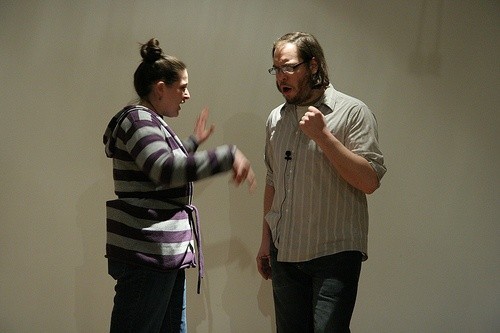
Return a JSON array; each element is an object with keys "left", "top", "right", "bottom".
[{"left": 268, "top": 60, "right": 308, "bottom": 75}]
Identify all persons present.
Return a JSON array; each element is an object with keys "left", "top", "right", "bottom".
[
  {"left": 103, "top": 38, "right": 257, "bottom": 333},
  {"left": 256, "top": 32, "right": 387, "bottom": 333}
]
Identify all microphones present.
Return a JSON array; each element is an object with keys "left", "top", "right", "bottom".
[{"left": 284, "top": 151, "right": 291, "bottom": 160}]
